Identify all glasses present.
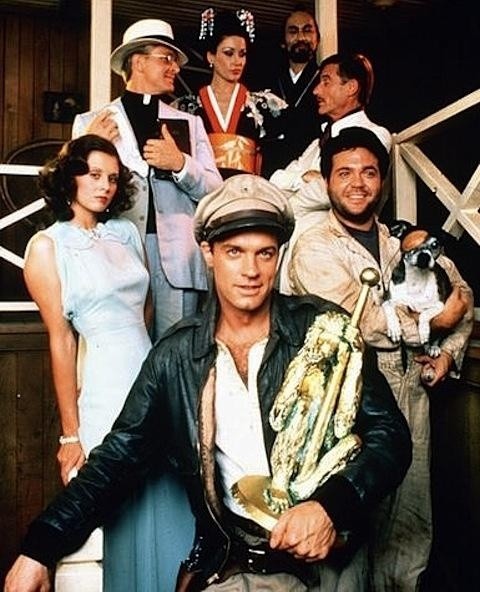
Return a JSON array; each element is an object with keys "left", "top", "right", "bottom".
[{"left": 151, "top": 53, "right": 174, "bottom": 62}]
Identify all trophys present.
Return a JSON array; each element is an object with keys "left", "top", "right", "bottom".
[{"left": 232, "top": 267, "right": 381, "bottom": 549}]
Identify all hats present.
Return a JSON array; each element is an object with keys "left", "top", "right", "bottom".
[
  {"left": 193, "top": 173, "right": 294, "bottom": 240},
  {"left": 109, "top": 19, "right": 188, "bottom": 73}
]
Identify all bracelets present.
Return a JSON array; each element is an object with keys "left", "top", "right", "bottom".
[{"left": 59, "top": 435, "right": 80, "bottom": 444}]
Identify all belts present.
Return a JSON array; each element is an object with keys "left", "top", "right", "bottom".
[{"left": 229, "top": 535, "right": 320, "bottom": 589}]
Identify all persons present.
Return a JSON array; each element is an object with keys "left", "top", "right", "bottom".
[
  {"left": 23, "top": 134, "right": 196, "bottom": 592},
  {"left": 263, "top": 10, "right": 333, "bottom": 180},
  {"left": 168, "top": 6, "right": 289, "bottom": 181},
  {"left": 71, "top": 19, "right": 225, "bottom": 343},
  {"left": 4, "top": 172, "right": 413, "bottom": 592},
  {"left": 288, "top": 128, "right": 474, "bottom": 592},
  {"left": 269, "top": 52, "right": 393, "bottom": 296}
]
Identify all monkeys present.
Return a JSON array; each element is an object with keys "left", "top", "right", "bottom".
[{"left": 264, "top": 310, "right": 365, "bottom": 516}]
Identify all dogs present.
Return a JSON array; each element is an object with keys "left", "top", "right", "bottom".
[{"left": 377, "top": 216, "right": 458, "bottom": 382}]
